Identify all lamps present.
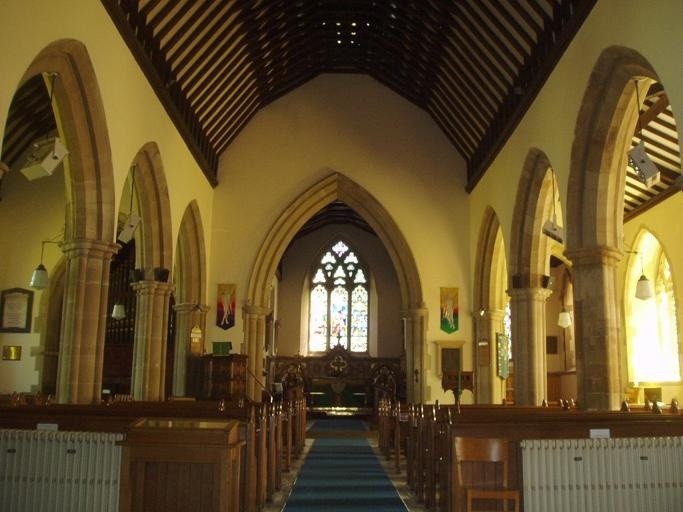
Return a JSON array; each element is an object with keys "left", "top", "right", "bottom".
[
  {"left": 191, "top": 304, "right": 205, "bottom": 343},
  {"left": 554, "top": 289, "right": 571, "bottom": 329},
  {"left": 29, "top": 240, "right": 63, "bottom": 290},
  {"left": 111, "top": 292, "right": 135, "bottom": 321},
  {"left": 626, "top": 79, "right": 661, "bottom": 187},
  {"left": 20, "top": 72, "right": 70, "bottom": 181},
  {"left": 625, "top": 250, "right": 653, "bottom": 300},
  {"left": 117, "top": 164, "right": 141, "bottom": 244},
  {"left": 542, "top": 168, "right": 564, "bottom": 244}
]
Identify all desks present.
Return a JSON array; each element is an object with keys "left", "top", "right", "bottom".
[{"left": 308, "top": 376, "right": 370, "bottom": 405}]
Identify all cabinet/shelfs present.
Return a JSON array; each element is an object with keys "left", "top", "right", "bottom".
[{"left": 200, "top": 354, "right": 247, "bottom": 398}]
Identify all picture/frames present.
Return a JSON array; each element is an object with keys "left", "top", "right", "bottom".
[{"left": 0, "top": 288, "right": 34, "bottom": 333}]
